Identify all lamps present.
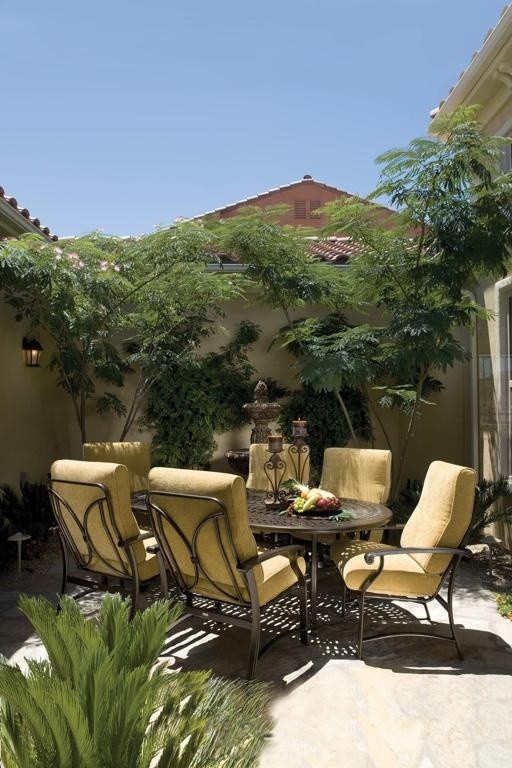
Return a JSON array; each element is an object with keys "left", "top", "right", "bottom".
[{"left": 21, "top": 320, "right": 43, "bottom": 368}]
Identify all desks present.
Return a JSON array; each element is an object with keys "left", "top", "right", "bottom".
[{"left": 128, "top": 488, "right": 393, "bottom": 635}]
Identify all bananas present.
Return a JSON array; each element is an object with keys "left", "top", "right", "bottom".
[{"left": 303, "top": 495, "right": 321, "bottom": 511}]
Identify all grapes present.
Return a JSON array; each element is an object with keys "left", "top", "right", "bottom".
[{"left": 294, "top": 498, "right": 305, "bottom": 510}]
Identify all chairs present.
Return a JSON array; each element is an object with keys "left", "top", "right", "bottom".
[
  {"left": 143, "top": 467, "right": 310, "bottom": 680},
  {"left": 45, "top": 459, "right": 180, "bottom": 624},
  {"left": 246, "top": 444, "right": 309, "bottom": 545},
  {"left": 329, "top": 459, "right": 479, "bottom": 663},
  {"left": 289, "top": 447, "right": 392, "bottom": 550},
  {"left": 81, "top": 441, "right": 152, "bottom": 493}
]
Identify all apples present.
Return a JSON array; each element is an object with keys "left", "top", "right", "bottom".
[{"left": 317, "top": 500, "right": 326, "bottom": 508}]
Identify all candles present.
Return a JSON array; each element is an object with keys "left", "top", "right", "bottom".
[
  {"left": 268, "top": 431, "right": 283, "bottom": 452},
  {"left": 292, "top": 417, "right": 308, "bottom": 436}
]
